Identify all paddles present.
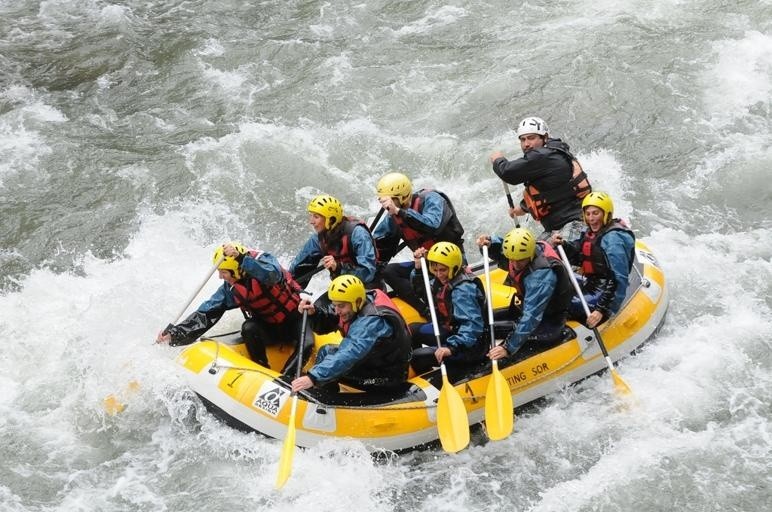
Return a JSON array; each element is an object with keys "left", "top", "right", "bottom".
[
  {"left": 275, "top": 301, "right": 311, "bottom": 492},
  {"left": 417, "top": 252, "right": 471, "bottom": 454},
  {"left": 553, "top": 236, "right": 634, "bottom": 396},
  {"left": 482, "top": 235, "right": 514, "bottom": 441},
  {"left": 102, "top": 244, "right": 235, "bottom": 419}
]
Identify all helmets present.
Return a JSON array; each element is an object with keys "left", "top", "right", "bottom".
[
  {"left": 518, "top": 117, "right": 550, "bottom": 138},
  {"left": 502, "top": 228, "right": 536, "bottom": 262},
  {"left": 582, "top": 192, "right": 614, "bottom": 226},
  {"left": 377, "top": 173, "right": 412, "bottom": 206},
  {"left": 328, "top": 275, "right": 366, "bottom": 312},
  {"left": 309, "top": 195, "right": 343, "bottom": 231},
  {"left": 427, "top": 242, "right": 462, "bottom": 278},
  {"left": 214, "top": 242, "right": 252, "bottom": 280}
]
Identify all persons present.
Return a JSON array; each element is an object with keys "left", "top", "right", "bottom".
[
  {"left": 476, "top": 227, "right": 574, "bottom": 360},
  {"left": 407, "top": 241, "right": 491, "bottom": 376},
  {"left": 157, "top": 242, "right": 316, "bottom": 378},
  {"left": 373, "top": 172, "right": 468, "bottom": 296},
  {"left": 490, "top": 117, "right": 592, "bottom": 252},
  {"left": 291, "top": 274, "right": 413, "bottom": 399},
  {"left": 549, "top": 191, "right": 635, "bottom": 329},
  {"left": 288, "top": 195, "right": 386, "bottom": 292}
]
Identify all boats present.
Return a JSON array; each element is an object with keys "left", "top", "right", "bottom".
[{"left": 170, "top": 241, "right": 666, "bottom": 459}]
